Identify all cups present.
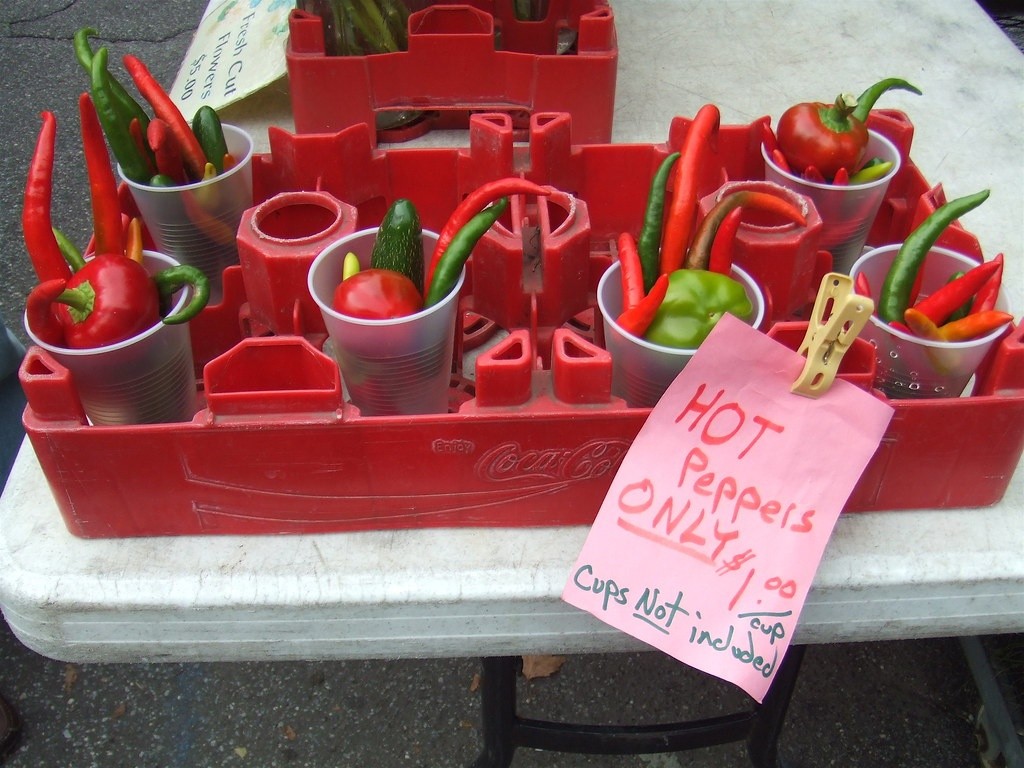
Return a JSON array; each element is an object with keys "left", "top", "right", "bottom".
[
  {"left": 23, "top": 249, "right": 198, "bottom": 426},
  {"left": 849, "top": 243, "right": 1012, "bottom": 398},
  {"left": 597, "top": 247, "right": 765, "bottom": 408},
  {"left": 306, "top": 227, "right": 466, "bottom": 417},
  {"left": 761, "top": 129, "right": 902, "bottom": 276}
]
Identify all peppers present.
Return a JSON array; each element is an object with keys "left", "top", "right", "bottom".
[
  {"left": 24, "top": 28, "right": 246, "bottom": 421},
  {"left": 608, "top": 75, "right": 1013, "bottom": 396},
  {"left": 327, "top": 176, "right": 551, "bottom": 412}
]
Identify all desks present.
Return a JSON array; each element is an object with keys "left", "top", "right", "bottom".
[{"left": 0, "top": 0, "right": 1024, "bottom": 766}]
